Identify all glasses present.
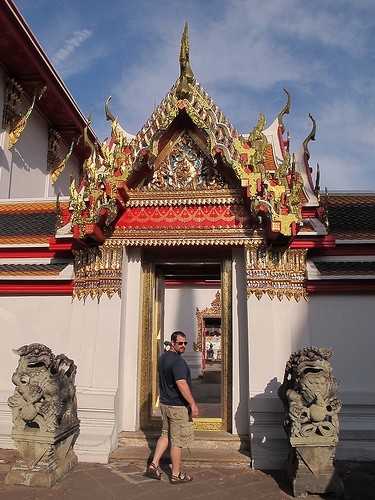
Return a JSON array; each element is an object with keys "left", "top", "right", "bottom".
[{"left": 175, "top": 341, "right": 188, "bottom": 346}]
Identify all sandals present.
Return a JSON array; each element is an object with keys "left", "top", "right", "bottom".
[
  {"left": 169, "top": 471, "right": 193, "bottom": 484},
  {"left": 146, "top": 461, "right": 161, "bottom": 481}
]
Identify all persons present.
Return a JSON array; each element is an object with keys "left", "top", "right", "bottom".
[{"left": 145, "top": 331, "right": 199, "bottom": 485}]
